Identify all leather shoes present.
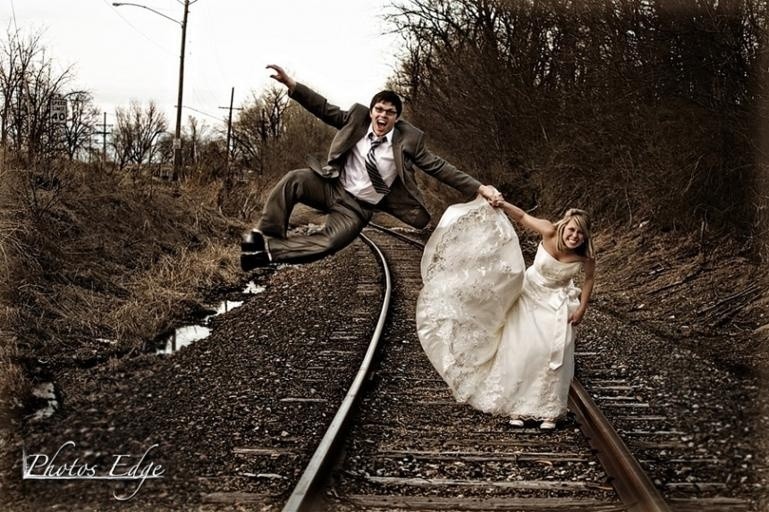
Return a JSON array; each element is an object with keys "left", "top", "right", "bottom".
[
  {"left": 240, "top": 231, "right": 264, "bottom": 252},
  {"left": 539, "top": 422, "right": 557, "bottom": 429},
  {"left": 508, "top": 419, "right": 524, "bottom": 427},
  {"left": 239, "top": 251, "right": 271, "bottom": 272}
]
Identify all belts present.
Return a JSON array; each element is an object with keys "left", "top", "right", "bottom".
[{"left": 344, "top": 190, "right": 377, "bottom": 211}]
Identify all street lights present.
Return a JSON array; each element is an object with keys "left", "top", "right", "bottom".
[
  {"left": 175, "top": 104, "right": 236, "bottom": 151},
  {"left": 112, "top": 0, "right": 191, "bottom": 178}
]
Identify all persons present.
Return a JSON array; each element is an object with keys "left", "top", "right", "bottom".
[
  {"left": 238, "top": 63, "right": 505, "bottom": 273},
  {"left": 482, "top": 183, "right": 596, "bottom": 430}
]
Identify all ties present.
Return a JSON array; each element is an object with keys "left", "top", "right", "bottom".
[{"left": 364, "top": 137, "right": 392, "bottom": 195}]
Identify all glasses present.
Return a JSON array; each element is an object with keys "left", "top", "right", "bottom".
[{"left": 372, "top": 106, "right": 399, "bottom": 116}]
